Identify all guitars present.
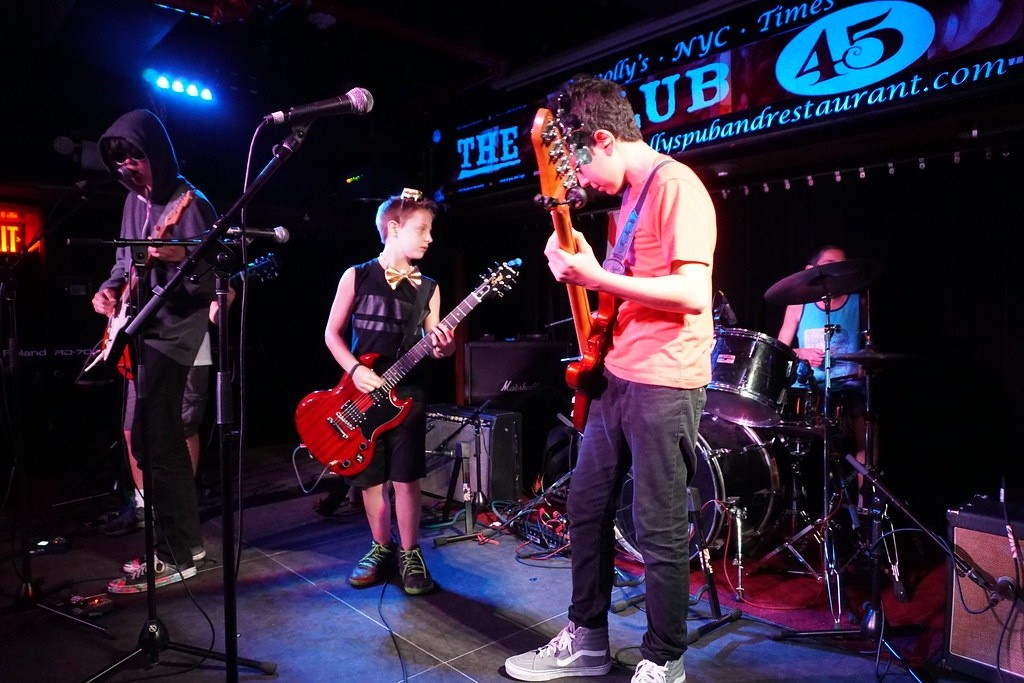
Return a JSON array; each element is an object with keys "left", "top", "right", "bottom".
[
  {"left": 532, "top": 107, "right": 618, "bottom": 431},
  {"left": 102, "top": 190, "right": 194, "bottom": 364},
  {"left": 296, "top": 259, "right": 523, "bottom": 477}
]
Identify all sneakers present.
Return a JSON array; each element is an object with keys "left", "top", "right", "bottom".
[
  {"left": 505, "top": 620, "right": 612, "bottom": 682},
  {"left": 398, "top": 544, "right": 433, "bottom": 594},
  {"left": 108, "top": 550, "right": 198, "bottom": 594},
  {"left": 630, "top": 655, "right": 687, "bottom": 683},
  {"left": 348, "top": 536, "right": 398, "bottom": 586},
  {"left": 122, "top": 543, "right": 206, "bottom": 573}
]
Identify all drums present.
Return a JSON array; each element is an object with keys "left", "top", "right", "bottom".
[
  {"left": 705, "top": 329, "right": 795, "bottom": 428},
  {"left": 779, "top": 387, "right": 848, "bottom": 435},
  {"left": 614, "top": 410, "right": 779, "bottom": 563}
]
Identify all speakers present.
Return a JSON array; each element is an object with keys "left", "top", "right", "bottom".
[
  {"left": 940, "top": 496, "right": 1024, "bottom": 683},
  {"left": 464, "top": 340, "right": 574, "bottom": 499},
  {"left": 417, "top": 403, "right": 522, "bottom": 513}
]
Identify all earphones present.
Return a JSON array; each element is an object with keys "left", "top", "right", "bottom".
[
  {"left": 604, "top": 137, "right": 611, "bottom": 147},
  {"left": 389, "top": 226, "right": 396, "bottom": 233}
]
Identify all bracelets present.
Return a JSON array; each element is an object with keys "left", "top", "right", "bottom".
[{"left": 350, "top": 363, "right": 363, "bottom": 379}]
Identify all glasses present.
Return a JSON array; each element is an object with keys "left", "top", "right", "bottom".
[{"left": 110, "top": 145, "right": 146, "bottom": 166}]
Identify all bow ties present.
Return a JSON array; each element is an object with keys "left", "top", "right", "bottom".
[{"left": 385, "top": 265, "right": 422, "bottom": 291}]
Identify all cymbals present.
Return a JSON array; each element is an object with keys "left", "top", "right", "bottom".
[
  {"left": 831, "top": 346, "right": 920, "bottom": 364},
  {"left": 765, "top": 259, "right": 889, "bottom": 305}
]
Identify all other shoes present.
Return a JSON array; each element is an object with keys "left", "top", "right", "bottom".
[{"left": 104, "top": 508, "right": 146, "bottom": 536}]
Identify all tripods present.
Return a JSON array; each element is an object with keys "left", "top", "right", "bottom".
[
  {"left": 0, "top": 190, "right": 277, "bottom": 683},
  {"left": 744, "top": 282, "right": 923, "bottom": 683}
]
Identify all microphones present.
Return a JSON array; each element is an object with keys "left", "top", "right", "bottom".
[
  {"left": 71, "top": 166, "right": 134, "bottom": 192},
  {"left": 425, "top": 450, "right": 458, "bottom": 459},
  {"left": 989, "top": 576, "right": 1017, "bottom": 607},
  {"left": 719, "top": 290, "right": 738, "bottom": 325},
  {"left": 227, "top": 226, "right": 289, "bottom": 244},
  {"left": 263, "top": 87, "right": 374, "bottom": 125}
]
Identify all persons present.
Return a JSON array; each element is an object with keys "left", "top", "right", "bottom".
[
  {"left": 776, "top": 245, "right": 879, "bottom": 512},
  {"left": 91, "top": 109, "right": 236, "bottom": 593},
  {"left": 324, "top": 194, "right": 456, "bottom": 596},
  {"left": 505, "top": 76, "right": 717, "bottom": 683}
]
